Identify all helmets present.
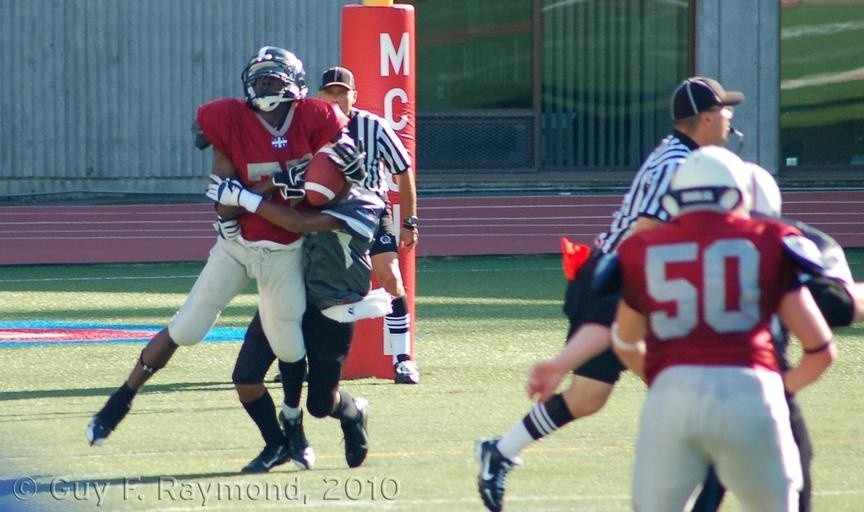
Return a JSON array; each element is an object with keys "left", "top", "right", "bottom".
[
  {"left": 658, "top": 146, "right": 752, "bottom": 216},
  {"left": 241, "top": 46, "right": 309, "bottom": 113},
  {"left": 747, "top": 162, "right": 782, "bottom": 218}
]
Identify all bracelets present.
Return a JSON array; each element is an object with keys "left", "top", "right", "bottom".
[
  {"left": 401, "top": 223, "right": 418, "bottom": 231},
  {"left": 402, "top": 216, "right": 420, "bottom": 226}
]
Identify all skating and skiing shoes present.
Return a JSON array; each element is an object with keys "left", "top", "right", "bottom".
[{"left": 343, "top": 397, "right": 367, "bottom": 468}]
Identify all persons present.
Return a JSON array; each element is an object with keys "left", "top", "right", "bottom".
[
  {"left": 475, "top": 75, "right": 745, "bottom": 511},
  {"left": 610, "top": 143, "right": 840, "bottom": 512},
  {"left": 272, "top": 65, "right": 422, "bottom": 384},
  {"left": 204, "top": 170, "right": 388, "bottom": 475},
  {"left": 692, "top": 161, "right": 864, "bottom": 512},
  {"left": 84, "top": 44, "right": 365, "bottom": 469}
]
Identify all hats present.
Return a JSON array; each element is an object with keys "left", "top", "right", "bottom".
[
  {"left": 320, "top": 67, "right": 354, "bottom": 92},
  {"left": 671, "top": 77, "right": 740, "bottom": 124}
]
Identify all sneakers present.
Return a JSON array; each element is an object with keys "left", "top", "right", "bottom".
[
  {"left": 84, "top": 391, "right": 133, "bottom": 446},
  {"left": 239, "top": 442, "right": 288, "bottom": 474},
  {"left": 278, "top": 408, "right": 315, "bottom": 471},
  {"left": 393, "top": 360, "right": 418, "bottom": 384}
]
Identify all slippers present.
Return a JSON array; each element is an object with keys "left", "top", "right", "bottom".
[{"left": 480, "top": 439, "right": 522, "bottom": 512}]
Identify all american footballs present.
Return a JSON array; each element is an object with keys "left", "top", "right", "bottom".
[{"left": 304, "top": 143, "right": 345, "bottom": 205}]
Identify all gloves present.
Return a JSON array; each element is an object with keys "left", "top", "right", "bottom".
[
  {"left": 214, "top": 220, "right": 241, "bottom": 239},
  {"left": 204, "top": 173, "right": 263, "bottom": 215}
]
